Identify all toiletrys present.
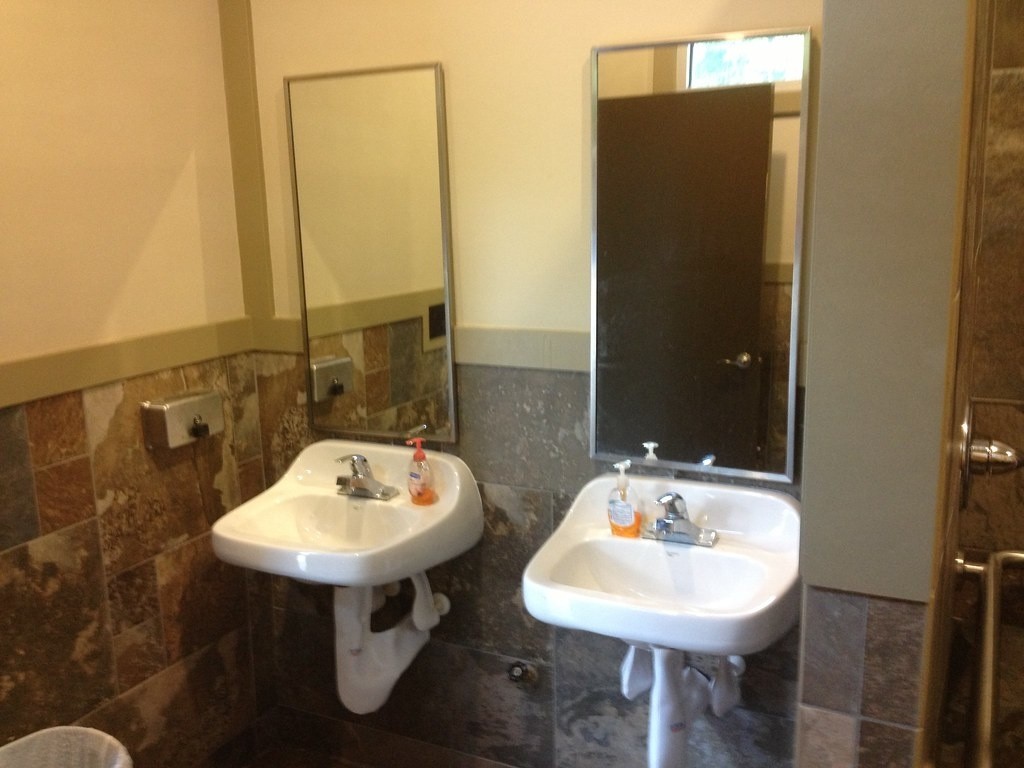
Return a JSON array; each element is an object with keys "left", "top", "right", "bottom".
[
  {"left": 406, "top": 438, "right": 433, "bottom": 507},
  {"left": 607, "top": 459, "right": 643, "bottom": 538}
]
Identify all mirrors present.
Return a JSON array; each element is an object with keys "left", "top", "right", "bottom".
[
  {"left": 588, "top": 26, "right": 812, "bottom": 483},
  {"left": 283, "top": 64, "right": 461, "bottom": 445}
]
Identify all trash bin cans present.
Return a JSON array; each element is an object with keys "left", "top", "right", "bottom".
[{"left": 0, "top": 725, "right": 134, "bottom": 768}]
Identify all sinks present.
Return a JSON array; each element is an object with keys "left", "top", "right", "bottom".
[
  {"left": 522, "top": 471, "right": 806, "bottom": 656},
  {"left": 212, "top": 439, "right": 485, "bottom": 586}
]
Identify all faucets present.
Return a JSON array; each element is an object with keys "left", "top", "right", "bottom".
[
  {"left": 651, "top": 492, "right": 691, "bottom": 535},
  {"left": 333, "top": 454, "right": 375, "bottom": 492}
]
